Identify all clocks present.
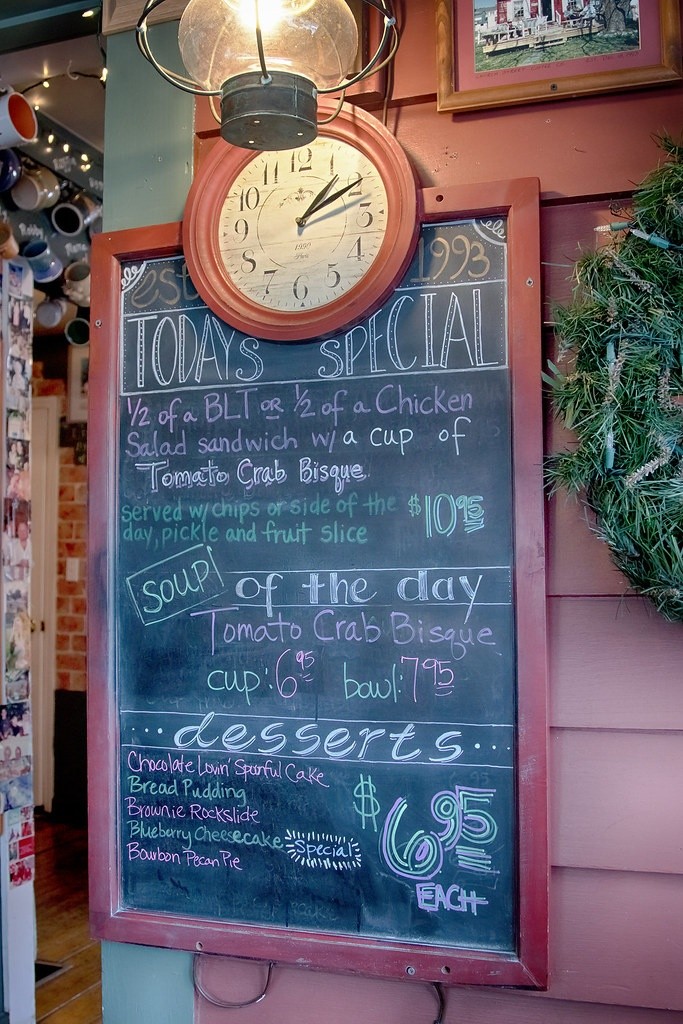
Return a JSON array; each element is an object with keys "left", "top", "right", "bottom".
[{"left": 183, "top": 97, "right": 417, "bottom": 341}]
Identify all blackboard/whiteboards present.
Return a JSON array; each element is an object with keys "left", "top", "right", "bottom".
[{"left": 88, "top": 178, "right": 548, "bottom": 991}]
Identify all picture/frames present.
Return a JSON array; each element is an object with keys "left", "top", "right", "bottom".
[{"left": 435, "top": 0, "right": 683, "bottom": 112}]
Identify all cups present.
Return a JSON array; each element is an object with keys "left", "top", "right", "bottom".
[
  {"left": 0, "top": 148, "right": 22, "bottom": 194},
  {"left": 50, "top": 189, "right": 103, "bottom": 237},
  {"left": 64, "top": 317, "right": 90, "bottom": 346},
  {"left": 0, "top": 85, "right": 38, "bottom": 150},
  {"left": 34, "top": 293, "right": 67, "bottom": 326},
  {"left": 0, "top": 223, "right": 20, "bottom": 259},
  {"left": 11, "top": 157, "right": 61, "bottom": 212},
  {"left": 62, "top": 262, "right": 91, "bottom": 307},
  {"left": 23, "top": 235, "right": 63, "bottom": 283}
]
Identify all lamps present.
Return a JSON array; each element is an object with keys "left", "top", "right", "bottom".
[{"left": 137, "top": 0, "right": 407, "bottom": 149}]
